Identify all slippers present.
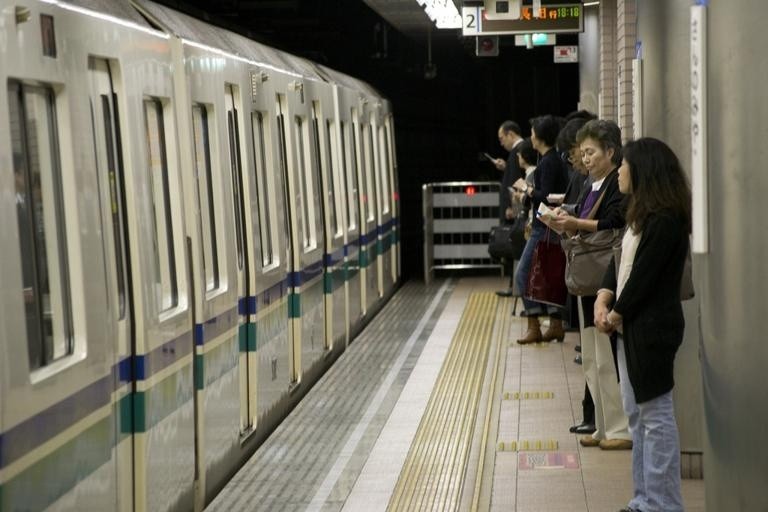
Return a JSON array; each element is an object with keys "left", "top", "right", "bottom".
[{"left": 542, "top": 316, "right": 565, "bottom": 343}]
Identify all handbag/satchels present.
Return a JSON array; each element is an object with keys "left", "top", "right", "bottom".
[
  {"left": 526, "top": 220, "right": 567, "bottom": 310},
  {"left": 563, "top": 184, "right": 695, "bottom": 303},
  {"left": 488, "top": 213, "right": 526, "bottom": 259}
]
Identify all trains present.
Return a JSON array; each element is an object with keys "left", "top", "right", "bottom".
[{"left": 0, "top": 1, "right": 402, "bottom": 511}]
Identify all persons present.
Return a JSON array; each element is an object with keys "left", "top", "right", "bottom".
[
  {"left": 594, "top": 138, "right": 692, "bottom": 510},
  {"left": 513, "top": 110, "right": 598, "bottom": 433},
  {"left": 550, "top": 119, "right": 633, "bottom": 450},
  {"left": 489, "top": 119, "right": 536, "bottom": 298}
]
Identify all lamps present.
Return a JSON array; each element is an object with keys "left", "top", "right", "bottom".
[{"left": 416, "top": 0, "right": 462, "bottom": 29}]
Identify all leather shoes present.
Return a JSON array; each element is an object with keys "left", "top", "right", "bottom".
[
  {"left": 494, "top": 288, "right": 513, "bottom": 298},
  {"left": 569, "top": 421, "right": 632, "bottom": 451}
]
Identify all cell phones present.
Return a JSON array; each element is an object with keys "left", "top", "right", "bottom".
[{"left": 485, "top": 153, "right": 497, "bottom": 163}]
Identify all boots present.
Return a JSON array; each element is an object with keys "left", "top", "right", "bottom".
[{"left": 518, "top": 314, "right": 543, "bottom": 345}]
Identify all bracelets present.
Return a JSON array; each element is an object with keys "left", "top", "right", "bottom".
[{"left": 607, "top": 315, "right": 622, "bottom": 325}]
